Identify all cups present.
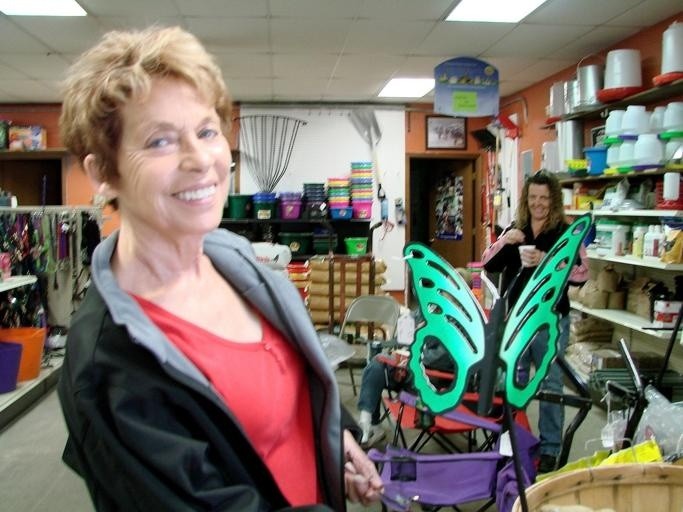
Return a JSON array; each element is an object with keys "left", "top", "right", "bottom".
[{"left": 518, "top": 246, "right": 536, "bottom": 267}]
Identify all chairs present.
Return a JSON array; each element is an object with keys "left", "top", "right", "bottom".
[{"left": 338, "top": 294, "right": 399, "bottom": 396}]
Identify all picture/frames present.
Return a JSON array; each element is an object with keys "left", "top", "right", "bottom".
[{"left": 425, "top": 114, "right": 467, "bottom": 151}]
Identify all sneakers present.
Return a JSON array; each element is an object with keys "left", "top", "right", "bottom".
[
  {"left": 359, "top": 423, "right": 386, "bottom": 450},
  {"left": 539, "top": 454, "right": 557, "bottom": 473}
]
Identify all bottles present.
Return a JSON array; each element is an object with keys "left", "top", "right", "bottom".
[
  {"left": 632, "top": 224, "right": 665, "bottom": 260},
  {"left": 396, "top": 307, "right": 417, "bottom": 344},
  {"left": 493, "top": 188, "right": 505, "bottom": 211}
]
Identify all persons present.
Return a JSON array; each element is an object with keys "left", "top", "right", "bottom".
[
  {"left": 356, "top": 267, "right": 475, "bottom": 449},
  {"left": 482, "top": 167, "right": 589, "bottom": 475},
  {"left": 57, "top": 29, "right": 385, "bottom": 512}
]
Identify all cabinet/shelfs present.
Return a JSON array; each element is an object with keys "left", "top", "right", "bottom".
[
  {"left": 538, "top": 76, "right": 683, "bottom": 341},
  {"left": 218, "top": 217, "right": 370, "bottom": 305}
]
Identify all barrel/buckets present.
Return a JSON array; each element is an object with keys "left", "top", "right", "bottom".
[
  {"left": 576, "top": 52, "right": 604, "bottom": 106},
  {"left": 0, "top": 340, "right": 23, "bottom": 395},
  {"left": 0, "top": 327, "right": 50, "bottom": 380},
  {"left": 582, "top": 146, "right": 608, "bottom": 178},
  {"left": 512, "top": 463, "right": 683, "bottom": 512},
  {"left": 344, "top": 237, "right": 368, "bottom": 257}
]
